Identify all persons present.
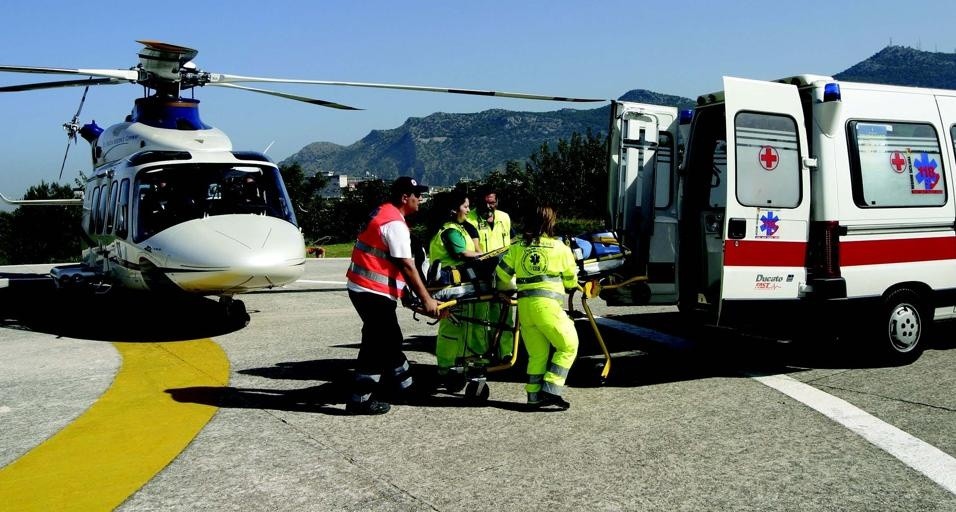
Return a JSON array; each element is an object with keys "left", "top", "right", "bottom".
[
  {"left": 428, "top": 187, "right": 578, "bottom": 411},
  {"left": 234, "top": 177, "right": 266, "bottom": 214},
  {"left": 347, "top": 177, "right": 446, "bottom": 416},
  {"left": 148, "top": 181, "right": 180, "bottom": 229}
]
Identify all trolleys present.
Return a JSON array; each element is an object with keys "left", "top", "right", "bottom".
[{"left": 400, "top": 242, "right": 649, "bottom": 404}]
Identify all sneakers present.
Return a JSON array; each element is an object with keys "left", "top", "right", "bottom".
[
  {"left": 538, "top": 390, "right": 569, "bottom": 410},
  {"left": 346, "top": 400, "right": 391, "bottom": 415}
]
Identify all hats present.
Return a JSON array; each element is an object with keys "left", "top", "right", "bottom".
[{"left": 390, "top": 176, "right": 429, "bottom": 197}]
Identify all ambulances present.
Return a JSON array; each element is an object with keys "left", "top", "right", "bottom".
[{"left": 601, "top": 75, "right": 955, "bottom": 365}]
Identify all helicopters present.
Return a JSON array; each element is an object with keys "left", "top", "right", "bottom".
[{"left": 0, "top": 38, "right": 613, "bottom": 328}]
